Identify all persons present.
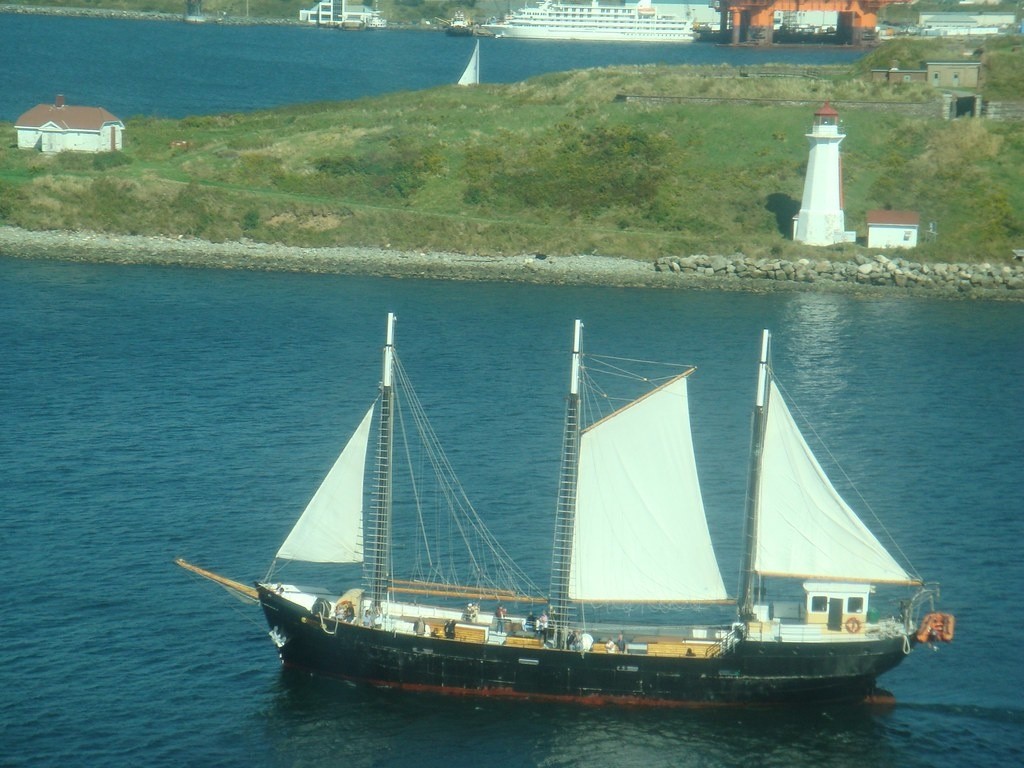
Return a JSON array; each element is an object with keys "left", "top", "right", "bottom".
[
  {"left": 414, "top": 617, "right": 425, "bottom": 636},
  {"left": 567, "top": 629, "right": 581, "bottom": 648},
  {"left": 497, "top": 605, "right": 507, "bottom": 634},
  {"left": 616, "top": 635, "right": 625, "bottom": 651},
  {"left": 540, "top": 611, "right": 547, "bottom": 642},
  {"left": 466, "top": 602, "right": 479, "bottom": 623},
  {"left": 605, "top": 639, "right": 615, "bottom": 653},
  {"left": 363, "top": 610, "right": 371, "bottom": 626},
  {"left": 345, "top": 602, "right": 355, "bottom": 622},
  {"left": 444, "top": 619, "right": 456, "bottom": 639}
]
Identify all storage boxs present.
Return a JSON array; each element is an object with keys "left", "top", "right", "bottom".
[{"left": 453, "top": 620, "right": 779, "bottom": 658}]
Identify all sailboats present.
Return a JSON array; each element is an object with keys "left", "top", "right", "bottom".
[
  {"left": 458, "top": 39, "right": 480, "bottom": 85},
  {"left": 174, "top": 312, "right": 955, "bottom": 710}
]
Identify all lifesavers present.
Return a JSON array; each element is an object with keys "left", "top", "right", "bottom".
[
  {"left": 845, "top": 617, "right": 862, "bottom": 634},
  {"left": 335, "top": 600, "right": 353, "bottom": 624}
]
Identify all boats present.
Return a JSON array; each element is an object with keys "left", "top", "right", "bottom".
[
  {"left": 481, "top": 0, "right": 700, "bottom": 42},
  {"left": 447, "top": 11, "right": 470, "bottom": 33}
]
